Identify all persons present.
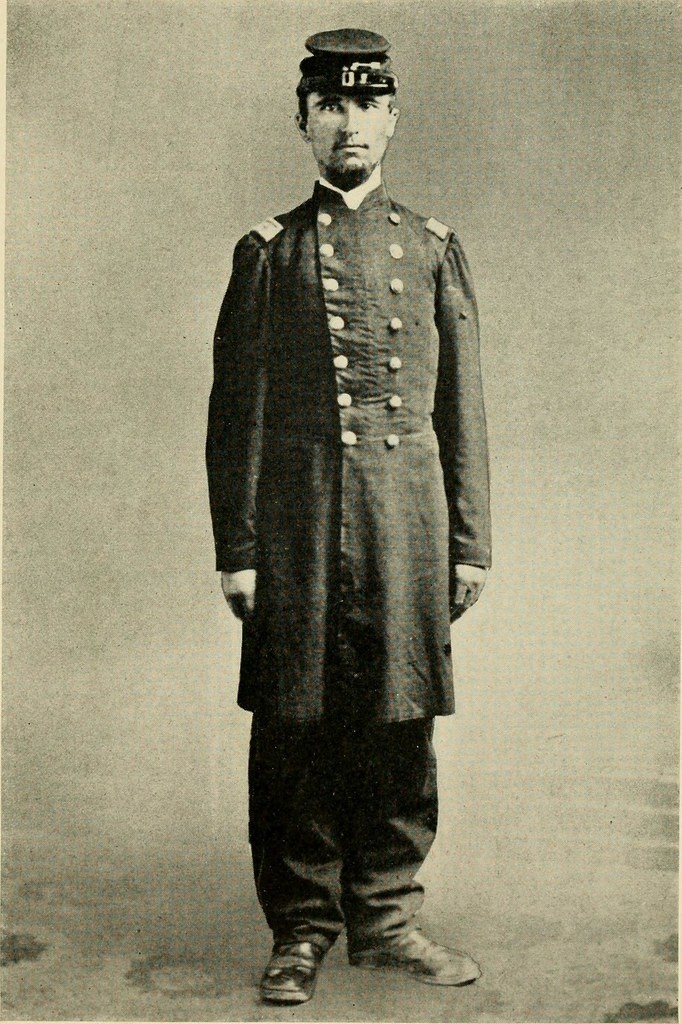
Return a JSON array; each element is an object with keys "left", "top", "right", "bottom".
[{"left": 206, "top": 30, "right": 491, "bottom": 1003}]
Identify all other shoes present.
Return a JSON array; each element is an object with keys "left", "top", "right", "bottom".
[
  {"left": 260, "top": 942, "right": 322, "bottom": 1003},
  {"left": 349, "top": 930, "right": 482, "bottom": 985}
]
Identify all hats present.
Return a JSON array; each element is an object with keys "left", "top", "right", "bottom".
[{"left": 298, "top": 28, "right": 398, "bottom": 96}]
